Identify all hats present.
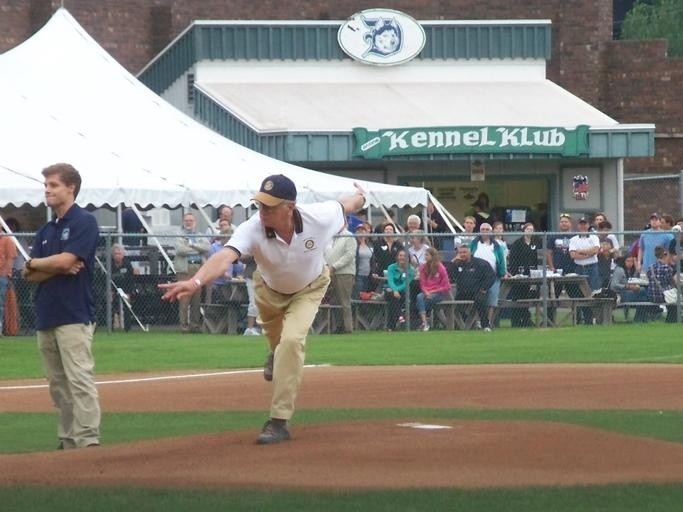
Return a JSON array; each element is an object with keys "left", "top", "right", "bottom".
[
  {"left": 356, "top": 224, "right": 366, "bottom": 231},
  {"left": 249, "top": 174, "right": 297, "bottom": 207},
  {"left": 650, "top": 213, "right": 658, "bottom": 219},
  {"left": 578, "top": 217, "right": 587, "bottom": 223}
]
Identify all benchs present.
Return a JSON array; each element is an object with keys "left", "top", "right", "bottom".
[{"left": 188, "top": 275, "right": 683, "bottom": 336}]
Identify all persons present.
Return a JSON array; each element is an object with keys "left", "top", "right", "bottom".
[
  {"left": 105, "top": 243, "right": 134, "bottom": 332},
  {"left": 311, "top": 191, "right": 682, "bottom": 333},
  {"left": 0, "top": 217, "right": 17, "bottom": 335},
  {"left": 23, "top": 163, "right": 102, "bottom": 448},
  {"left": 176, "top": 205, "right": 261, "bottom": 335},
  {"left": 5, "top": 218, "right": 31, "bottom": 280},
  {"left": 156, "top": 174, "right": 368, "bottom": 445}
]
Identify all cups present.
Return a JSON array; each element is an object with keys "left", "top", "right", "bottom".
[
  {"left": 517, "top": 265, "right": 563, "bottom": 279},
  {"left": 383, "top": 270, "right": 388, "bottom": 277},
  {"left": 629, "top": 273, "right": 648, "bottom": 283}
]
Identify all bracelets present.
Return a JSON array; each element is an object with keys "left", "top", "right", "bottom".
[{"left": 25, "top": 258, "right": 31, "bottom": 271}]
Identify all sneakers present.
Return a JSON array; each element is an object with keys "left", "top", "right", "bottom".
[
  {"left": 417, "top": 321, "right": 431, "bottom": 331},
  {"left": 256, "top": 420, "right": 289, "bottom": 443},
  {"left": 244, "top": 328, "right": 260, "bottom": 336},
  {"left": 263, "top": 356, "right": 273, "bottom": 381}
]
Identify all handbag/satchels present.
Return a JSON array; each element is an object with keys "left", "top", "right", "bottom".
[
  {"left": 663, "top": 288, "right": 683, "bottom": 304},
  {"left": 593, "top": 288, "right": 618, "bottom": 303},
  {"left": 621, "top": 286, "right": 649, "bottom": 302}
]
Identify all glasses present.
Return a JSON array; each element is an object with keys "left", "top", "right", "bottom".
[{"left": 559, "top": 214, "right": 569, "bottom": 218}]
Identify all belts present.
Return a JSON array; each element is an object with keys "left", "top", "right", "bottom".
[{"left": 188, "top": 260, "right": 199, "bottom": 264}]
